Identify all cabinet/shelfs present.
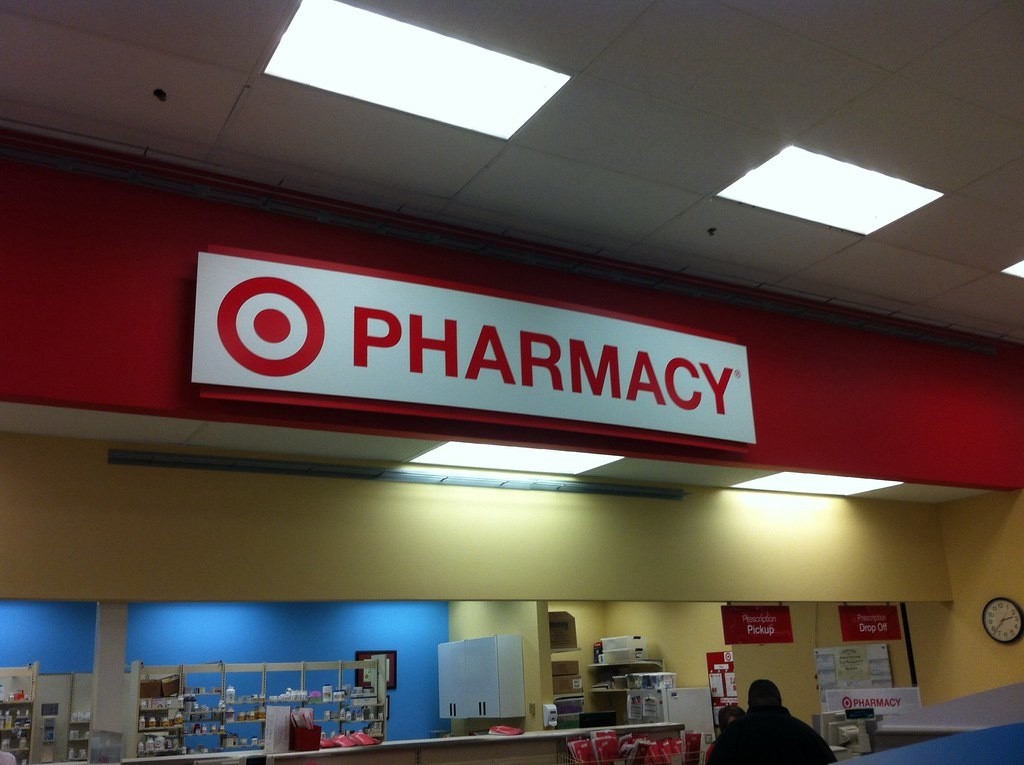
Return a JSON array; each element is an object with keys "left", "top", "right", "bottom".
[
  {"left": 0, "top": 660, "right": 41, "bottom": 765},
  {"left": 587, "top": 659, "right": 665, "bottom": 693},
  {"left": 438, "top": 633, "right": 526, "bottom": 719}
]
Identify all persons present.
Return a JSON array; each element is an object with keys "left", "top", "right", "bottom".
[
  {"left": 707, "top": 679, "right": 838, "bottom": 765},
  {"left": 704, "top": 705, "right": 745, "bottom": 765}
]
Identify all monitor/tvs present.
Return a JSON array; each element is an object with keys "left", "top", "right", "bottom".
[
  {"left": 819, "top": 710, "right": 859, "bottom": 747},
  {"left": 579, "top": 710, "right": 617, "bottom": 729}
]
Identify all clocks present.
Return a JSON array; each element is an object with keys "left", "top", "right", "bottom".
[{"left": 982, "top": 597, "right": 1023, "bottom": 644}]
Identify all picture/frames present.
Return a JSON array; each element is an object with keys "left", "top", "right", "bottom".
[
  {"left": 355, "top": 650, "right": 397, "bottom": 690},
  {"left": 350, "top": 695, "right": 390, "bottom": 721}
]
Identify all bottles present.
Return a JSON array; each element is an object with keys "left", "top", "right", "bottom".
[
  {"left": 194, "top": 723, "right": 207, "bottom": 734},
  {"left": 268, "top": 688, "right": 308, "bottom": 700},
  {"left": 71, "top": 712, "right": 90, "bottom": 723},
  {"left": 68, "top": 748, "right": 74, "bottom": 759},
  {"left": 225, "top": 708, "right": 235, "bottom": 722},
  {"left": 237, "top": 706, "right": 266, "bottom": 721},
  {"left": 238, "top": 694, "right": 265, "bottom": 703},
  {"left": 239, "top": 737, "right": 248, "bottom": 745},
  {"left": 138, "top": 741, "right": 144, "bottom": 754},
  {"left": 221, "top": 732, "right": 238, "bottom": 746},
  {"left": 14, "top": 709, "right": 31, "bottom": 728},
  {"left": 594, "top": 642, "right": 603, "bottom": 664},
  {"left": 160, "top": 717, "right": 169, "bottom": 727},
  {"left": 139, "top": 716, "right": 146, "bottom": 728},
  {"left": 181, "top": 746, "right": 186, "bottom": 755},
  {"left": 187, "top": 745, "right": 208, "bottom": 753},
  {"left": 69, "top": 730, "right": 80, "bottom": 740},
  {"left": 154, "top": 736, "right": 179, "bottom": 749},
  {"left": 0, "top": 710, "right": 12, "bottom": 729},
  {"left": 322, "top": 684, "right": 332, "bottom": 703},
  {"left": 194, "top": 688, "right": 205, "bottom": 694},
  {"left": 324, "top": 705, "right": 374, "bottom": 720},
  {"left": 174, "top": 712, "right": 183, "bottom": 724},
  {"left": 149, "top": 717, "right": 156, "bottom": 727},
  {"left": 191, "top": 701, "right": 226, "bottom": 721},
  {"left": 212, "top": 686, "right": 222, "bottom": 693},
  {"left": 333, "top": 690, "right": 346, "bottom": 701},
  {"left": 2, "top": 739, "right": 10, "bottom": 750},
  {"left": 145, "top": 737, "right": 154, "bottom": 750},
  {"left": 79, "top": 749, "right": 86, "bottom": 758},
  {"left": 352, "top": 686, "right": 373, "bottom": 694},
  {"left": 219, "top": 725, "right": 224, "bottom": 734},
  {"left": 210, "top": 725, "right": 217, "bottom": 734},
  {"left": 251, "top": 736, "right": 257, "bottom": 746},
  {"left": 226, "top": 685, "right": 235, "bottom": 703},
  {"left": 0, "top": 685, "right": 5, "bottom": 702},
  {"left": 19, "top": 737, "right": 27, "bottom": 748}
]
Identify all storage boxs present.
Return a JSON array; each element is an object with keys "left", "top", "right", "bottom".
[
  {"left": 290, "top": 725, "right": 322, "bottom": 752},
  {"left": 600, "top": 635, "right": 647, "bottom": 663},
  {"left": 140, "top": 673, "right": 179, "bottom": 698},
  {"left": 552, "top": 660, "right": 583, "bottom": 694}
]
